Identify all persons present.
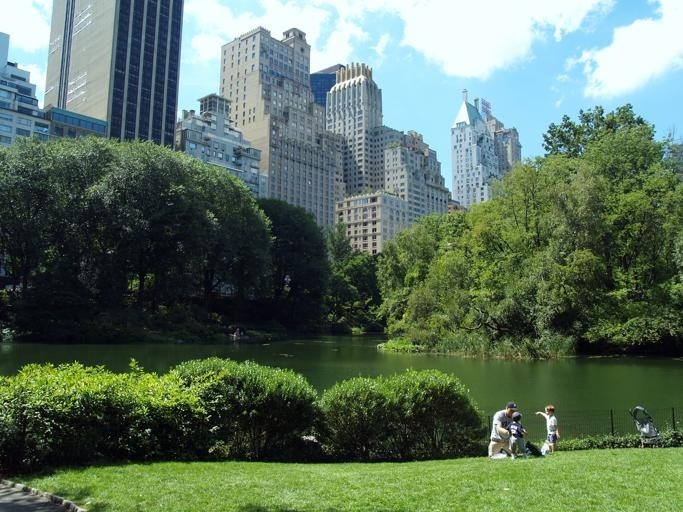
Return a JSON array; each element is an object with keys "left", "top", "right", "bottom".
[
  {"left": 506, "top": 412, "right": 532, "bottom": 460},
  {"left": 488, "top": 399, "right": 519, "bottom": 459},
  {"left": 535, "top": 403, "right": 561, "bottom": 454}
]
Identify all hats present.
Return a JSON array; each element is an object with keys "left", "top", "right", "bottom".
[
  {"left": 506, "top": 402, "right": 519, "bottom": 410},
  {"left": 512, "top": 412, "right": 522, "bottom": 421}
]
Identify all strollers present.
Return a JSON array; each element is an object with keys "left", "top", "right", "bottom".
[{"left": 629, "top": 406, "right": 665, "bottom": 448}]
